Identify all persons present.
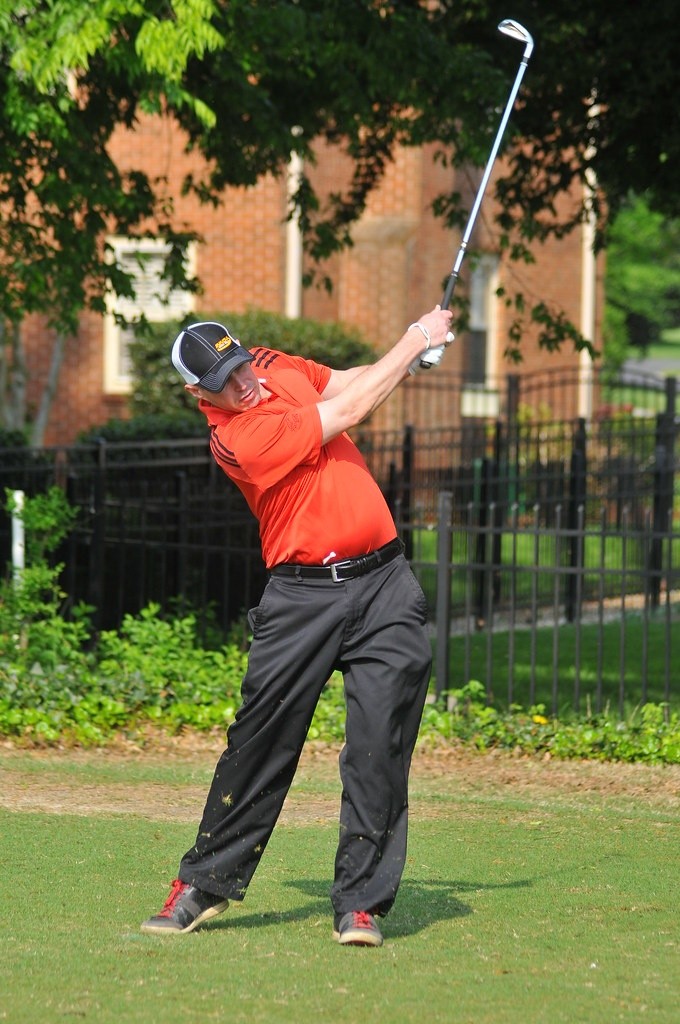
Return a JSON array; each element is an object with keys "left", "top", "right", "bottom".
[{"left": 137, "top": 305, "right": 453, "bottom": 935}]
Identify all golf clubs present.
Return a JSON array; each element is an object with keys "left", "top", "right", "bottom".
[{"left": 418, "top": 18, "right": 535, "bottom": 370}]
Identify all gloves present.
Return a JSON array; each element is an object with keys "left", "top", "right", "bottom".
[{"left": 407, "top": 331, "right": 454, "bottom": 376}]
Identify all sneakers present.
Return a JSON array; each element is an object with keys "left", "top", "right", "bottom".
[
  {"left": 140, "top": 880, "right": 229, "bottom": 935},
  {"left": 332, "top": 910, "right": 383, "bottom": 947}
]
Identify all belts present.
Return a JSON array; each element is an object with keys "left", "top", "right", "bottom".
[{"left": 271, "top": 536, "right": 405, "bottom": 583}]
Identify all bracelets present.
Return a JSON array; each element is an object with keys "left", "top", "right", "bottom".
[{"left": 409, "top": 322, "right": 431, "bottom": 352}]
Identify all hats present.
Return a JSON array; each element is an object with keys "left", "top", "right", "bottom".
[{"left": 171, "top": 322, "right": 255, "bottom": 393}]
